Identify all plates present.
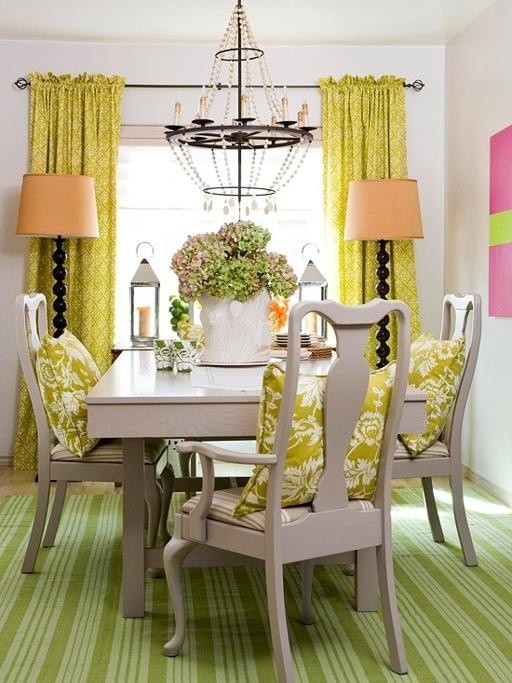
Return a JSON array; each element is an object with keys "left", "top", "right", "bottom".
[{"left": 274, "top": 333, "right": 311, "bottom": 348}]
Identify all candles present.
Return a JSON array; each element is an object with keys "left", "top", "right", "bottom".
[
  {"left": 309, "top": 311, "right": 317, "bottom": 333},
  {"left": 139, "top": 307, "right": 152, "bottom": 336}
]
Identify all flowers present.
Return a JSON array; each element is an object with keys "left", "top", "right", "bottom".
[{"left": 170, "top": 222, "right": 299, "bottom": 302}]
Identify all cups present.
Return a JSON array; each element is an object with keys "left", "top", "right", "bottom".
[
  {"left": 173, "top": 339, "right": 204, "bottom": 372},
  {"left": 153, "top": 339, "right": 177, "bottom": 374}
]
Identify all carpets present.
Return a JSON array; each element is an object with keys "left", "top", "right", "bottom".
[{"left": 0, "top": 485, "right": 512, "bottom": 683}]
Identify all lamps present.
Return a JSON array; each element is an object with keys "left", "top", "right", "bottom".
[
  {"left": 165, "top": 0, "right": 317, "bottom": 221},
  {"left": 344, "top": 178, "right": 424, "bottom": 369},
  {"left": 16, "top": 173, "right": 99, "bottom": 338}
]
